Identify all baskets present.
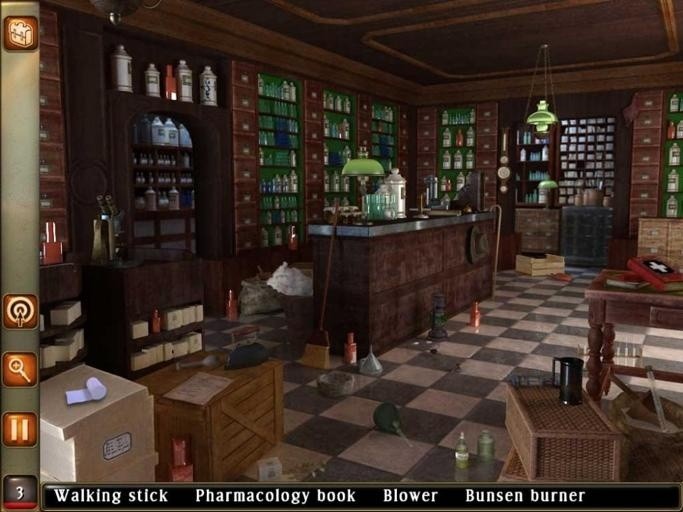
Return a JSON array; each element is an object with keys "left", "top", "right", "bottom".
[{"left": 498, "top": 376, "right": 624, "bottom": 484}]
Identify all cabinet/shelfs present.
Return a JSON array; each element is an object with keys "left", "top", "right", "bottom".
[
  {"left": 515, "top": 208, "right": 560, "bottom": 254},
  {"left": 561, "top": 205, "right": 614, "bottom": 267},
  {"left": 627, "top": 90, "right": 664, "bottom": 238},
  {"left": 40, "top": 350, "right": 285, "bottom": 483},
  {"left": 106, "top": 89, "right": 232, "bottom": 259},
  {"left": 41, "top": 42, "right": 67, "bottom": 251},
  {"left": 637, "top": 216, "right": 683, "bottom": 270},
  {"left": 662, "top": 86, "right": 683, "bottom": 219},
  {"left": 40, "top": 263, "right": 89, "bottom": 382},
  {"left": 82, "top": 258, "right": 204, "bottom": 381},
  {"left": 552, "top": 114, "right": 615, "bottom": 207},
  {"left": 514, "top": 121, "right": 554, "bottom": 208}
]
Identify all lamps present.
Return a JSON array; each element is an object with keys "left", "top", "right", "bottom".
[{"left": 523, "top": 44, "right": 560, "bottom": 133}]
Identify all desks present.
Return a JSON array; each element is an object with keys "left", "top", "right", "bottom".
[{"left": 584, "top": 268, "right": 683, "bottom": 410}]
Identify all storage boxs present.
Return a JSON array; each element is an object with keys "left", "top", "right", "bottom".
[
  {"left": 500, "top": 376, "right": 622, "bottom": 482},
  {"left": 495, "top": 445, "right": 530, "bottom": 483}
]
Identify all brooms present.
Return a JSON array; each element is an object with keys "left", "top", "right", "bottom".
[{"left": 303, "top": 195, "right": 340, "bottom": 370}]
